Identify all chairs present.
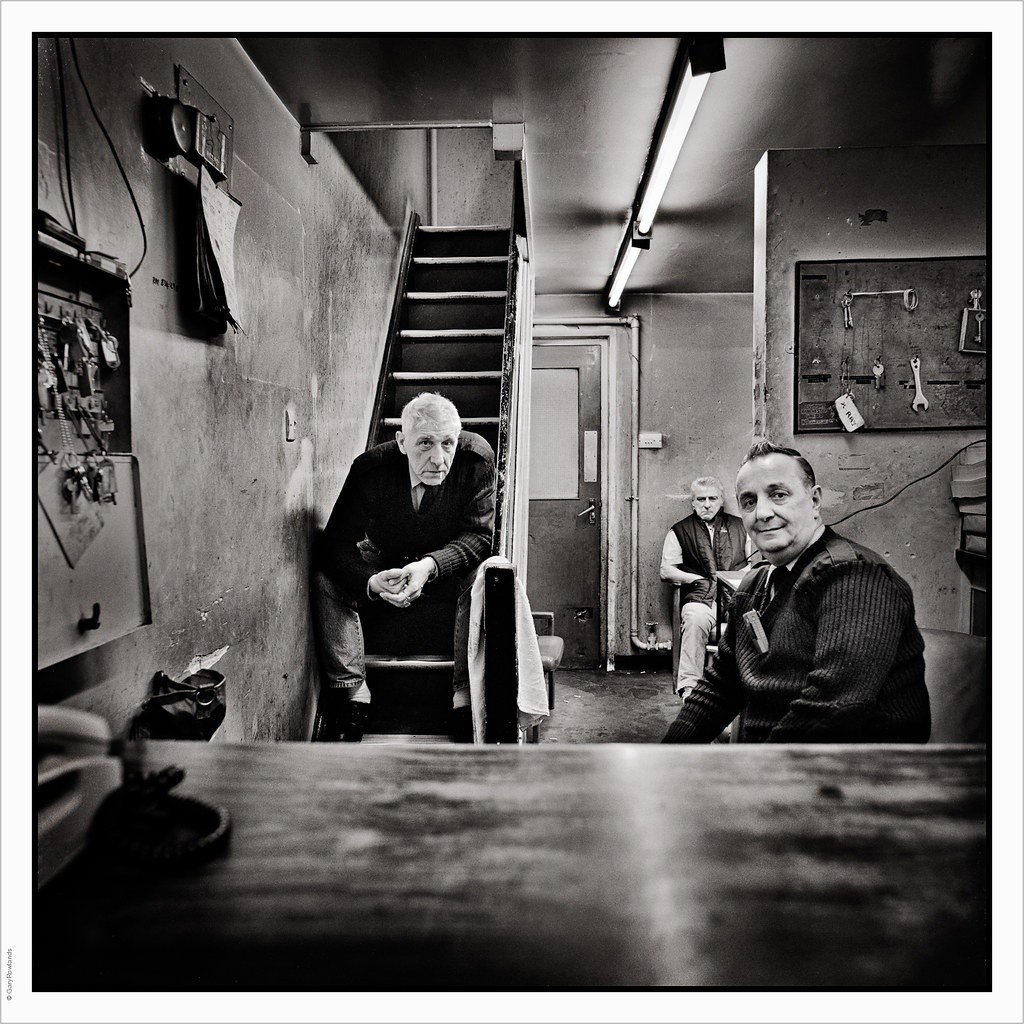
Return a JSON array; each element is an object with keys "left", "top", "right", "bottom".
[{"left": 672, "top": 580, "right": 722, "bottom": 694}]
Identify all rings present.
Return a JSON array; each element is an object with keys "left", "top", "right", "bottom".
[{"left": 403, "top": 602, "right": 411, "bottom": 608}]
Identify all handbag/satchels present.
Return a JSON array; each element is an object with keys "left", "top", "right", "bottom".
[{"left": 139, "top": 668, "right": 226, "bottom": 742}]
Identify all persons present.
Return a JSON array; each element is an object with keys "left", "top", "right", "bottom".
[
  {"left": 311, "top": 393, "right": 494, "bottom": 744},
  {"left": 660, "top": 477, "right": 752, "bottom": 705},
  {"left": 659, "top": 439, "right": 931, "bottom": 745}
]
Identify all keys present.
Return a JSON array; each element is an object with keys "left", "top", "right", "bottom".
[
  {"left": 974, "top": 313, "right": 985, "bottom": 342},
  {"left": 63, "top": 465, "right": 117, "bottom": 514},
  {"left": 842, "top": 394, "right": 855, "bottom": 431},
  {"left": 841, "top": 299, "right": 853, "bottom": 328},
  {"left": 38, "top": 429, "right": 59, "bottom": 466},
  {"left": 872, "top": 365, "right": 884, "bottom": 389},
  {"left": 99, "top": 420, "right": 114, "bottom": 451}
]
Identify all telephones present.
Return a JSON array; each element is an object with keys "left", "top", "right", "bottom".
[{"left": 34, "top": 701, "right": 125, "bottom": 894}]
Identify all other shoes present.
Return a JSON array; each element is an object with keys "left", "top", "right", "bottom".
[
  {"left": 682, "top": 689, "right": 691, "bottom": 703},
  {"left": 343, "top": 698, "right": 372, "bottom": 741},
  {"left": 447, "top": 705, "right": 473, "bottom": 743}
]
